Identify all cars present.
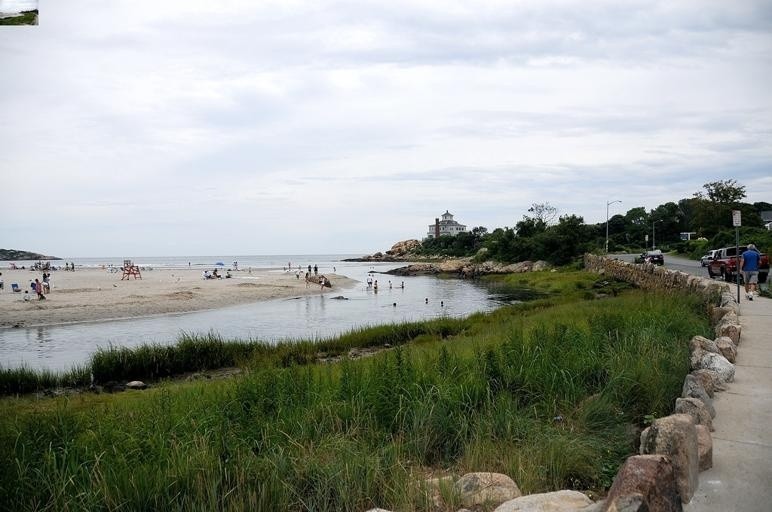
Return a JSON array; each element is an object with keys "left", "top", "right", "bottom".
[
  {"left": 641, "top": 250, "right": 664, "bottom": 265},
  {"left": 700, "top": 250, "right": 717, "bottom": 266}
]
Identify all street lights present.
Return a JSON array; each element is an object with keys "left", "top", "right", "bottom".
[
  {"left": 606, "top": 200, "right": 621, "bottom": 253},
  {"left": 653, "top": 220, "right": 663, "bottom": 249}
]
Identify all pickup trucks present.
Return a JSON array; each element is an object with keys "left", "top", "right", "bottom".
[{"left": 707, "top": 246, "right": 770, "bottom": 283}]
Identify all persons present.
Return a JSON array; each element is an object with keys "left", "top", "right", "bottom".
[
  {"left": 295, "top": 264, "right": 332, "bottom": 291},
  {"left": 21, "top": 259, "right": 76, "bottom": 303},
  {"left": 388, "top": 280, "right": 392, "bottom": 289},
  {"left": 212, "top": 269, "right": 221, "bottom": 278},
  {"left": 739, "top": 243, "right": 762, "bottom": 301},
  {"left": 0, "top": 272, "right": 5, "bottom": 295},
  {"left": 373, "top": 280, "right": 377, "bottom": 290},
  {"left": 232, "top": 261, "right": 235, "bottom": 270},
  {"left": 225, "top": 271, "right": 232, "bottom": 278},
  {"left": 643, "top": 254, "right": 652, "bottom": 264},
  {"left": 400, "top": 281, "right": 405, "bottom": 289},
  {"left": 234, "top": 261, "right": 239, "bottom": 270},
  {"left": 366, "top": 274, "right": 373, "bottom": 287},
  {"left": 203, "top": 270, "right": 212, "bottom": 279}
]
